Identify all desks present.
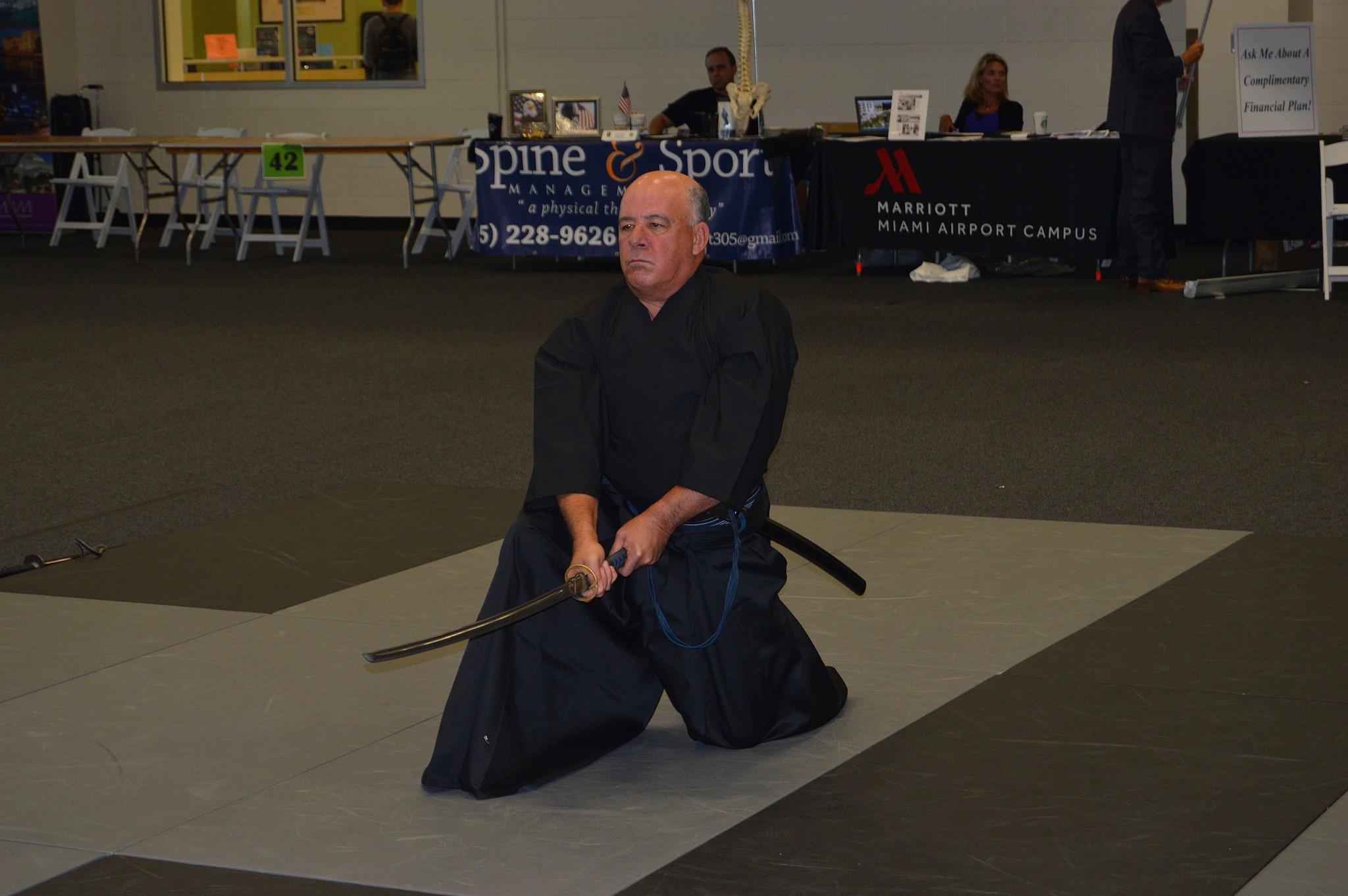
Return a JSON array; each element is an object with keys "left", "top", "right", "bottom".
[
  {"left": 0, "top": 134, "right": 197, "bottom": 266},
  {"left": 160, "top": 133, "right": 474, "bottom": 270},
  {"left": 183, "top": 55, "right": 363, "bottom": 71},
  {"left": 466, "top": 125, "right": 1348, "bottom": 287}
]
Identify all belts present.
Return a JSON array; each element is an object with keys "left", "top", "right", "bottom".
[{"left": 688, "top": 483, "right": 866, "bottom": 596}]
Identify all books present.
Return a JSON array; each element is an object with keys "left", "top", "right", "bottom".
[{"left": 653, "top": 134, "right": 676, "bottom": 138}]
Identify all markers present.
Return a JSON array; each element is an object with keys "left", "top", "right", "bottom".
[{"left": 1009, "top": 134, "right": 1031, "bottom": 137}]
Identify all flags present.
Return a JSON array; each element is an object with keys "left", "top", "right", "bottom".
[
  {"left": 511, "top": 94, "right": 544, "bottom": 129},
  {"left": 573, "top": 102, "right": 595, "bottom": 129},
  {"left": 618, "top": 86, "right": 631, "bottom": 116}
]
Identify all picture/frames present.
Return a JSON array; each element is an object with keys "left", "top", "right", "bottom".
[
  {"left": 887, "top": 88, "right": 930, "bottom": 141},
  {"left": 506, "top": 87, "right": 547, "bottom": 137},
  {"left": 551, "top": 96, "right": 602, "bottom": 139},
  {"left": 853, "top": 95, "right": 893, "bottom": 136}
]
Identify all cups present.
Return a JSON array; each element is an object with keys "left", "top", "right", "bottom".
[
  {"left": 487, "top": 114, "right": 502, "bottom": 140},
  {"left": 630, "top": 114, "right": 646, "bottom": 132},
  {"left": 1033, "top": 111, "right": 1048, "bottom": 135},
  {"left": 612, "top": 112, "right": 630, "bottom": 130}
]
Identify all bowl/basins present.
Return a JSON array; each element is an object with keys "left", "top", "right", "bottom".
[{"left": 516, "top": 122, "right": 549, "bottom": 140}]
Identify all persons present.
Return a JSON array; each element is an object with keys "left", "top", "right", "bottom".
[
  {"left": 939, "top": 53, "right": 1025, "bottom": 136},
  {"left": 363, "top": 0, "right": 418, "bottom": 82},
  {"left": 1108, "top": 0, "right": 1205, "bottom": 291},
  {"left": 648, "top": 46, "right": 764, "bottom": 137},
  {"left": 720, "top": 107, "right": 729, "bottom": 136},
  {"left": 421, "top": 173, "right": 848, "bottom": 799}
]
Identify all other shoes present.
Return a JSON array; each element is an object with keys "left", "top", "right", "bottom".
[
  {"left": 1136, "top": 276, "right": 1185, "bottom": 293},
  {"left": 1120, "top": 273, "right": 1137, "bottom": 289}
]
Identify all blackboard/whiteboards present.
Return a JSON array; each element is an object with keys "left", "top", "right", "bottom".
[{"left": 254, "top": 24, "right": 318, "bottom": 71}]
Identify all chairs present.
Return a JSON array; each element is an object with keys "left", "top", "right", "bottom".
[
  {"left": 235, "top": 130, "right": 334, "bottom": 263},
  {"left": 1318, "top": 139, "right": 1348, "bottom": 303},
  {"left": 409, "top": 125, "right": 509, "bottom": 258},
  {"left": 156, "top": 126, "right": 249, "bottom": 251},
  {"left": 48, "top": 126, "right": 142, "bottom": 250}
]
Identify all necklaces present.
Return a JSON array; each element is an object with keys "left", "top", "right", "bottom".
[{"left": 984, "top": 101, "right": 999, "bottom": 111}]
